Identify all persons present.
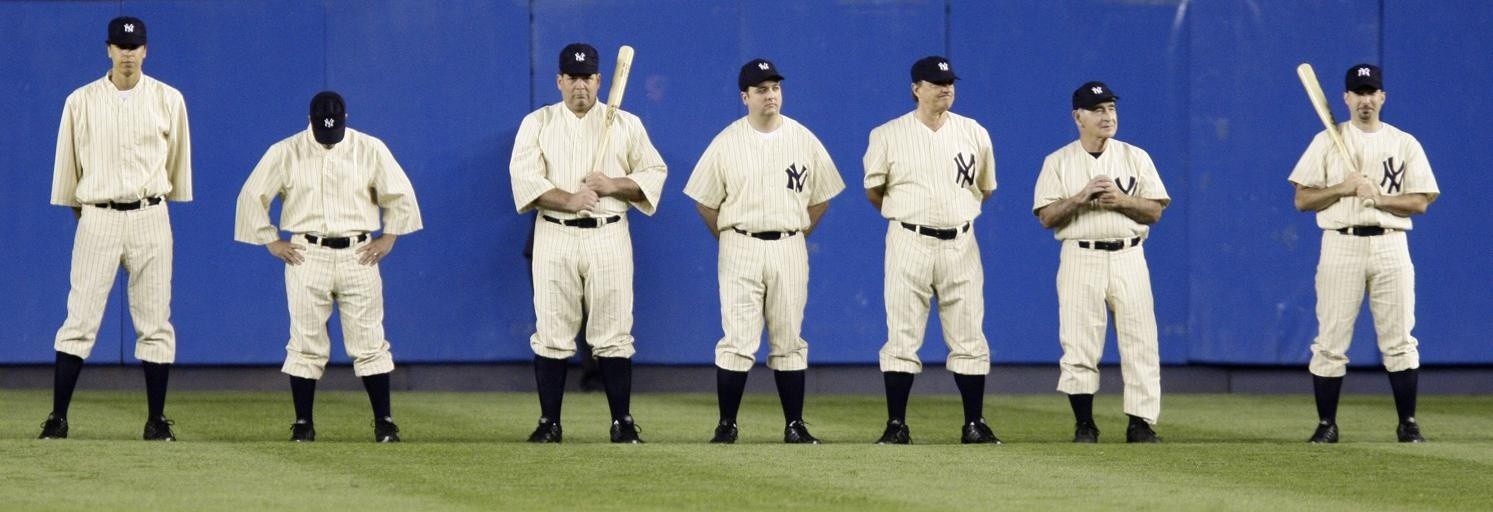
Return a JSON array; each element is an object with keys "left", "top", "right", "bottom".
[
  {"left": 230, "top": 92, "right": 424, "bottom": 442},
  {"left": 1032, "top": 81, "right": 1172, "bottom": 445},
  {"left": 681, "top": 58, "right": 846, "bottom": 444},
  {"left": 505, "top": 43, "right": 667, "bottom": 443},
  {"left": 1284, "top": 63, "right": 1440, "bottom": 443},
  {"left": 859, "top": 55, "right": 1002, "bottom": 445},
  {"left": 38, "top": 17, "right": 195, "bottom": 441}
]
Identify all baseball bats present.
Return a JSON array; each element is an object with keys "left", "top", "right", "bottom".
[
  {"left": 579, "top": 45, "right": 634, "bottom": 218},
  {"left": 1297, "top": 63, "right": 1373, "bottom": 208}
]
